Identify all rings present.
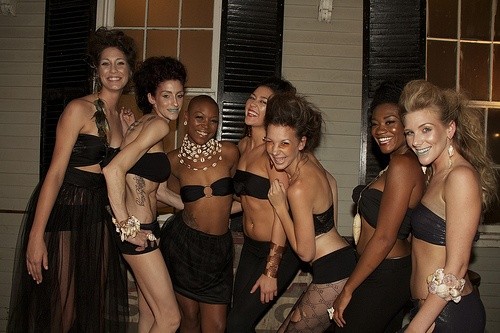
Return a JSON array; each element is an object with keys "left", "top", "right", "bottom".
[{"left": 327, "top": 306, "right": 335, "bottom": 320}]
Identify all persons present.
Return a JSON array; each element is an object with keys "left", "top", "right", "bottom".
[
  {"left": 223, "top": 78, "right": 302, "bottom": 333},
  {"left": 326, "top": 80, "right": 427, "bottom": 333},
  {"left": 264, "top": 95, "right": 360, "bottom": 333},
  {"left": 101, "top": 55, "right": 184, "bottom": 332},
  {"left": 395, "top": 79, "right": 500, "bottom": 333},
  {"left": 155, "top": 95, "right": 240, "bottom": 332},
  {"left": 7, "top": 26, "right": 140, "bottom": 333}
]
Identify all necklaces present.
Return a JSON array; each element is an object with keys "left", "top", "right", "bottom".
[
  {"left": 94, "top": 91, "right": 111, "bottom": 144},
  {"left": 352, "top": 165, "right": 390, "bottom": 245},
  {"left": 178, "top": 133, "right": 223, "bottom": 172}
]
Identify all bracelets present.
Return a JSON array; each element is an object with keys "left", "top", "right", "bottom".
[
  {"left": 263, "top": 242, "right": 284, "bottom": 279},
  {"left": 426, "top": 268, "right": 465, "bottom": 303},
  {"left": 110, "top": 215, "right": 140, "bottom": 242}
]
